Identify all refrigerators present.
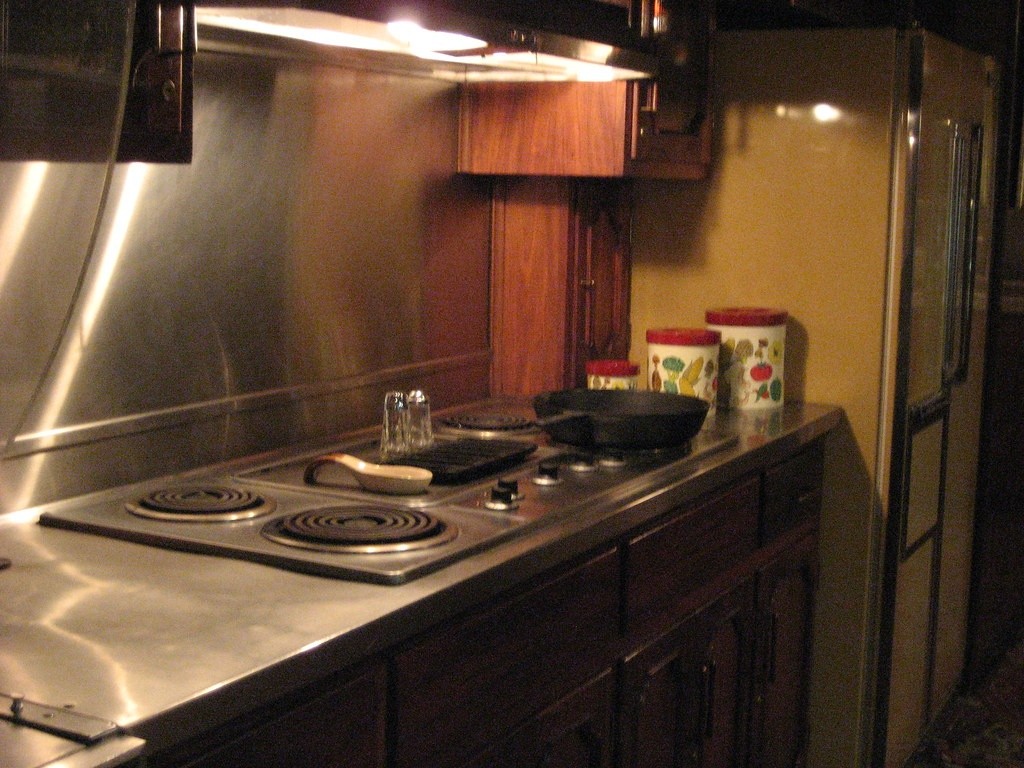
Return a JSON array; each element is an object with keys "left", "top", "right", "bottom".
[{"left": 629, "top": 22, "right": 1001, "bottom": 767}]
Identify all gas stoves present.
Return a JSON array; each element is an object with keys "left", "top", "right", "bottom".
[{"left": 37, "top": 391, "right": 739, "bottom": 583}]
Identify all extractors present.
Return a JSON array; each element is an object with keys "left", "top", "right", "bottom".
[{"left": 185, "top": 1, "right": 660, "bottom": 83}]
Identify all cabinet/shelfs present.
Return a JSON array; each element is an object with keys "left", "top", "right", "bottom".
[
  {"left": 175, "top": 436, "right": 824, "bottom": 768},
  {"left": 457, "top": 0, "right": 713, "bottom": 401}
]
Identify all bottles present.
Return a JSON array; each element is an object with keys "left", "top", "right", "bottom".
[
  {"left": 405, "top": 390, "right": 433, "bottom": 453},
  {"left": 379, "top": 391, "right": 409, "bottom": 460}
]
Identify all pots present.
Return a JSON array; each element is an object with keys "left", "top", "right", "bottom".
[{"left": 533, "top": 390, "right": 711, "bottom": 452}]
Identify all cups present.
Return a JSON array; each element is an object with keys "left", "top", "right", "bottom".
[
  {"left": 703, "top": 305, "right": 786, "bottom": 410},
  {"left": 584, "top": 360, "right": 639, "bottom": 391},
  {"left": 646, "top": 328, "right": 721, "bottom": 419}
]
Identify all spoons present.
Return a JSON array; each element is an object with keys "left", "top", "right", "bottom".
[{"left": 305, "top": 454, "right": 432, "bottom": 493}]
